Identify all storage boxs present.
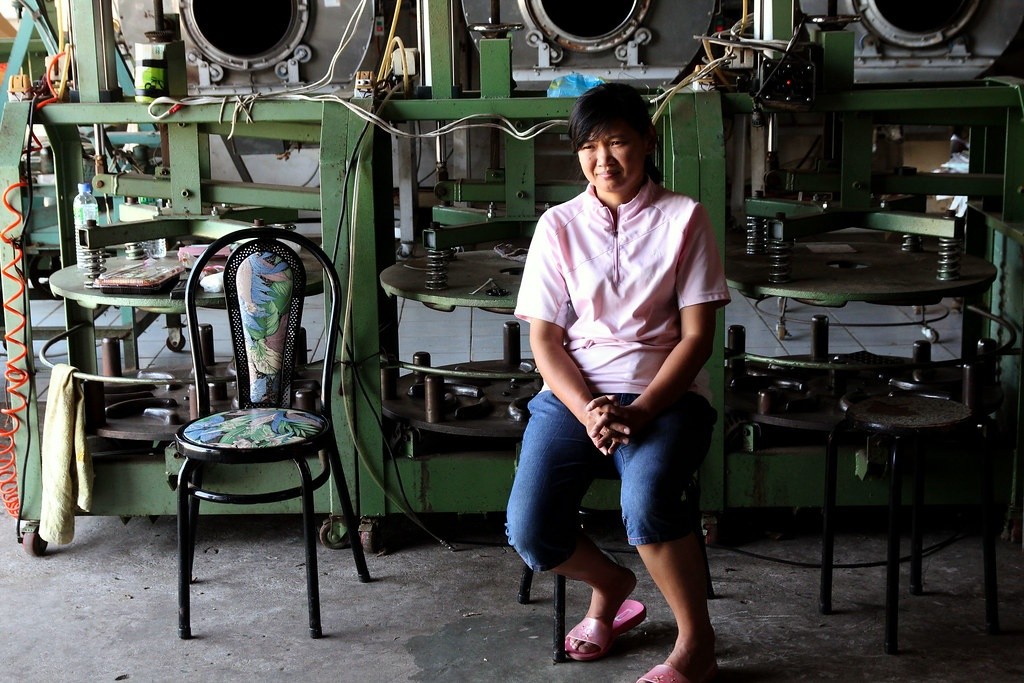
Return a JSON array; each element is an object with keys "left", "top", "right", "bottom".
[{"left": 91, "top": 264, "right": 187, "bottom": 296}]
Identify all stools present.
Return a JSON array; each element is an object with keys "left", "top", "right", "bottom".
[
  {"left": 507, "top": 395, "right": 715, "bottom": 665},
  {"left": 820, "top": 394, "right": 1001, "bottom": 655}
]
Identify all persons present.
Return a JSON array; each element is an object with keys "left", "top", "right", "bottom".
[{"left": 505, "top": 82, "right": 732, "bottom": 683}]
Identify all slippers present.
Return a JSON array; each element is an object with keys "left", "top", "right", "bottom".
[
  {"left": 564, "top": 599, "right": 647, "bottom": 661},
  {"left": 634, "top": 650, "right": 718, "bottom": 683}
]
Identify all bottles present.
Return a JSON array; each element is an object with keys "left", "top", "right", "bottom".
[
  {"left": 72, "top": 182, "right": 99, "bottom": 269},
  {"left": 138, "top": 196, "right": 166, "bottom": 259}
]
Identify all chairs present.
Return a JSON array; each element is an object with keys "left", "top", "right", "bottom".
[{"left": 177, "top": 227, "right": 371, "bottom": 638}]
